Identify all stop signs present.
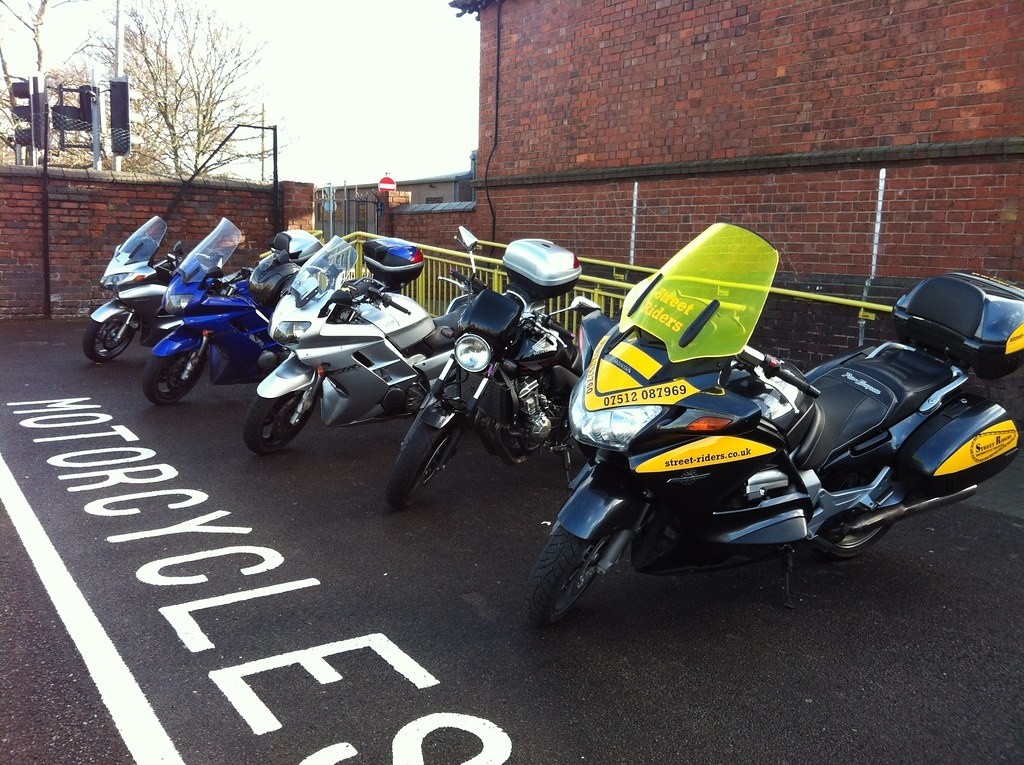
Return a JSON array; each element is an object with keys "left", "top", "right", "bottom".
[{"left": 377, "top": 176, "right": 398, "bottom": 193}]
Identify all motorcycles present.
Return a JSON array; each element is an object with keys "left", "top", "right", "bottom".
[
  {"left": 520, "top": 223, "right": 1023, "bottom": 630},
  {"left": 83, "top": 225, "right": 603, "bottom": 512}
]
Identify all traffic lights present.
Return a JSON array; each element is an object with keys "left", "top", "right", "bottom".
[{"left": 11, "top": 70, "right": 45, "bottom": 151}]
